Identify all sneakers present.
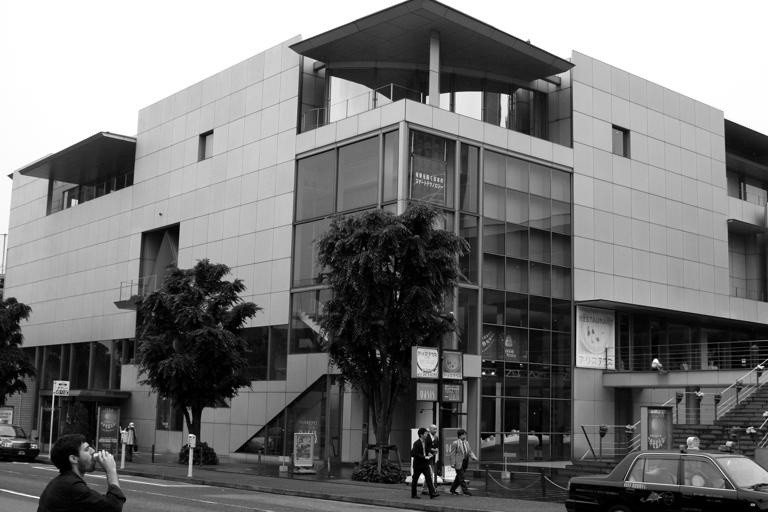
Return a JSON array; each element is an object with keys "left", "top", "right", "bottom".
[{"left": 410, "top": 490, "right": 474, "bottom": 499}]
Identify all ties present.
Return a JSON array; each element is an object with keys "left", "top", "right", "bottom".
[{"left": 462, "top": 442, "right": 466, "bottom": 459}]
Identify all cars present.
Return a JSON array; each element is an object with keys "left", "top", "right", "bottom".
[
  {"left": 565, "top": 449, "right": 768, "bottom": 512},
  {"left": 0, "top": 423, "right": 40, "bottom": 462}
]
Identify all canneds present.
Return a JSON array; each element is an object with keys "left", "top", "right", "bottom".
[{"left": 92, "top": 451, "right": 108, "bottom": 461}]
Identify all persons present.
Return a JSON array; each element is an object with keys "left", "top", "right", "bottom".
[
  {"left": 421, "top": 423, "right": 440, "bottom": 497},
  {"left": 691, "top": 462, "right": 724, "bottom": 487},
  {"left": 449, "top": 429, "right": 479, "bottom": 498},
  {"left": 37, "top": 431, "right": 127, "bottom": 511},
  {"left": 124, "top": 422, "right": 137, "bottom": 464},
  {"left": 749, "top": 343, "right": 761, "bottom": 369},
  {"left": 410, "top": 427, "right": 441, "bottom": 500},
  {"left": 651, "top": 355, "right": 663, "bottom": 371}
]
